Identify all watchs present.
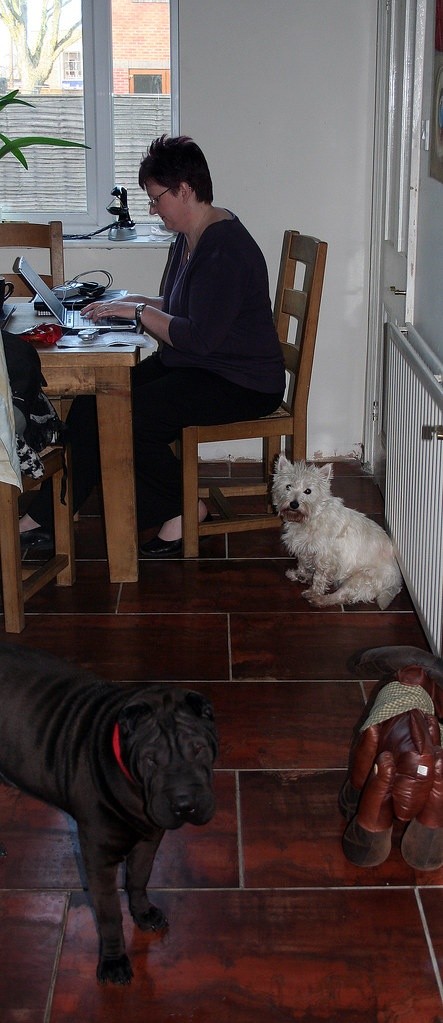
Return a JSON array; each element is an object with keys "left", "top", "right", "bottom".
[{"left": 136, "top": 302, "right": 147, "bottom": 327}]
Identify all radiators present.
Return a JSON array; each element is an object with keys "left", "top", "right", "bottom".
[{"left": 380, "top": 321, "right": 442, "bottom": 661}]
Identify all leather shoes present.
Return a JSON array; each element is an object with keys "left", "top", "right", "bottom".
[
  {"left": 142, "top": 513, "right": 215, "bottom": 557},
  {"left": 20, "top": 518, "right": 44, "bottom": 544}
]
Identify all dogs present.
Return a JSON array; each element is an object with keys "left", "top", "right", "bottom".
[
  {"left": 0, "top": 638, "right": 219, "bottom": 987},
  {"left": 271, "top": 455, "right": 404, "bottom": 608}
]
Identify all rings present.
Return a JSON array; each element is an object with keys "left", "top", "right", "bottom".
[{"left": 104, "top": 306, "right": 108, "bottom": 311}]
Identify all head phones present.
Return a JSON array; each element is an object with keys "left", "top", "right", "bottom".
[{"left": 73, "top": 268, "right": 114, "bottom": 298}]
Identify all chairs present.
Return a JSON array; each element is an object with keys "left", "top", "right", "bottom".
[
  {"left": 0, "top": 329, "right": 76, "bottom": 633},
  {"left": 170, "top": 229, "right": 328, "bottom": 557},
  {"left": 0, "top": 219, "right": 65, "bottom": 302}
]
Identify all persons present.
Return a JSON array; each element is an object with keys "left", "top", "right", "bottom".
[{"left": 0, "top": 134, "right": 287, "bottom": 558}]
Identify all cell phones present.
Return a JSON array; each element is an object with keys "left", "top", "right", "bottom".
[{"left": 79, "top": 328, "right": 100, "bottom": 343}]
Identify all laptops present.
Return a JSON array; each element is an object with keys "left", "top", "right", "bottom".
[
  {"left": 34, "top": 289, "right": 129, "bottom": 312},
  {"left": 18, "top": 256, "right": 136, "bottom": 334}
]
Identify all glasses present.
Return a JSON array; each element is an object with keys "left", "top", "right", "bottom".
[{"left": 149, "top": 184, "right": 178, "bottom": 206}]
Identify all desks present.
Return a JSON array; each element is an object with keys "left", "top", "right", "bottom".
[{"left": 0, "top": 295, "right": 145, "bottom": 583}]
